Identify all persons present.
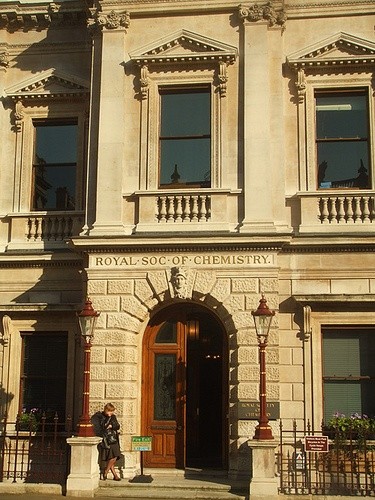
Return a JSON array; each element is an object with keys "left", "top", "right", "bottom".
[
  {"left": 170, "top": 267, "right": 189, "bottom": 298},
  {"left": 95, "top": 404, "right": 120, "bottom": 480}
]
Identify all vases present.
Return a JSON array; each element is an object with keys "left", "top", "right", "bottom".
[
  {"left": 18, "top": 421, "right": 35, "bottom": 431},
  {"left": 349, "top": 429, "right": 370, "bottom": 440},
  {"left": 330, "top": 428, "right": 349, "bottom": 439}
]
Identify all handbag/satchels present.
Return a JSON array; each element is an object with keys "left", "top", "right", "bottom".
[{"left": 99, "top": 430, "right": 116, "bottom": 449}]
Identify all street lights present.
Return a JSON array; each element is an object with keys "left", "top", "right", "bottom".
[
  {"left": 75, "top": 294, "right": 101, "bottom": 437},
  {"left": 251, "top": 293, "right": 275, "bottom": 439}
]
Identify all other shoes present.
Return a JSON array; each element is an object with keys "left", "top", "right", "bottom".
[
  {"left": 103, "top": 473, "right": 106, "bottom": 480},
  {"left": 113, "top": 477, "right": 121, "bottom": 481}
]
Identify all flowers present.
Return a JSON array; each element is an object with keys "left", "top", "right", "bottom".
[
  {"left": 327, "top": 412, "right": 350, "bottom": 431},
  {"left": 16, "top": 407, "right": 39, "bottom": 431},
  {"left": 349, "top": 413, "right": 375, "bottom": 434}
]
